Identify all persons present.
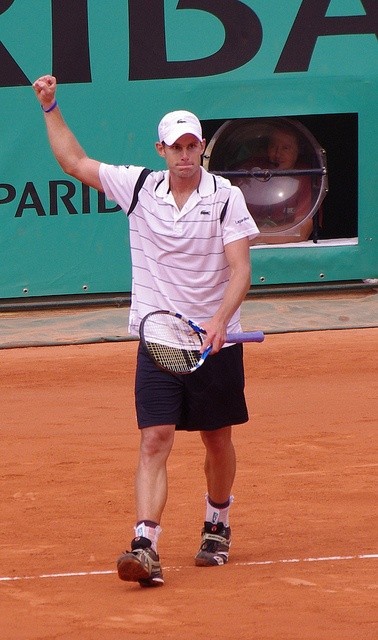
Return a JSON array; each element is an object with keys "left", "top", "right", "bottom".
[
  {"left": 230, "top": 129, "right": 324, "bottom": 244},
  {"left": 32, "top": 73, "right": 260, "bottom": 585}
]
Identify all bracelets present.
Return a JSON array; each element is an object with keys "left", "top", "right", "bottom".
[{"left": 40, "top": 100, "right": 58, "bottom": 112}]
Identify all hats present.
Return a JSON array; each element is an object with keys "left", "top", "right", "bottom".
[{"left": 157, "top": 109, "right": 204, "bottom": 146}]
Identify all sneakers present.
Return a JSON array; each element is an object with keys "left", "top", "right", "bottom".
[
  {"left": 117, "top": 537, "right": 164, "bottom": 587},
  {"left": 193, "top": 522, "right": 230, "bottom": 566}
]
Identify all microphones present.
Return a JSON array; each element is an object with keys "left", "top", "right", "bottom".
[{"left": 265, "top": 159, "right": 280, "bottom": 167}]
